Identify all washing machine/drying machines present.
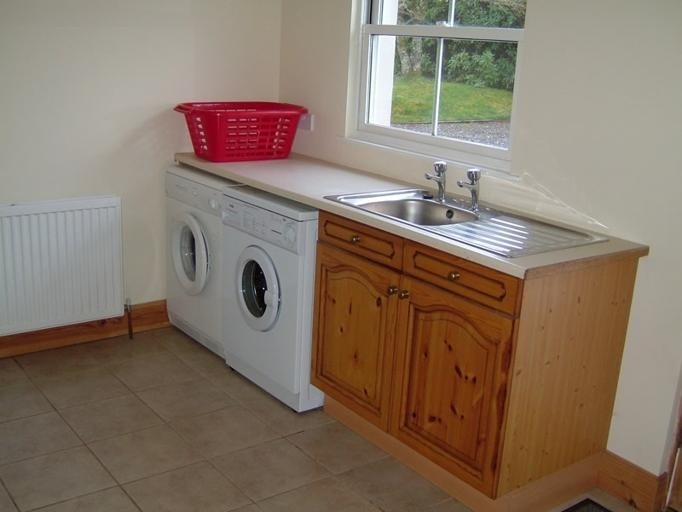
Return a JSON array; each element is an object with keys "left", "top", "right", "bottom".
[
  {"left": 165, "top": 166, "right": 246, "bottom": 361},
  {"left": 222, "top": 184, "right": 324, "bottom": 412}
]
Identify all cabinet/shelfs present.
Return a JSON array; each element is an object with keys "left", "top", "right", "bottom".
[{"left": 309, "top": 209, "right": 650, "bottom": 501}]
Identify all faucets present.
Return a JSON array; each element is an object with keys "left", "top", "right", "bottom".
[
  {"left": 425, "top": 161, "right": 447, "bottom": 201},
  {"left": 457, "top": 170, "right": 481, "bottom": 210}
]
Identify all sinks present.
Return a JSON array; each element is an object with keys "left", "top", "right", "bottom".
[{"left": 356, "top": 197, "right": 479, "bottom": 227}]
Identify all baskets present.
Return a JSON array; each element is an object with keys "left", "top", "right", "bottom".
[{"left": 172, "top": 100, "right": 309, "bottom": 163}]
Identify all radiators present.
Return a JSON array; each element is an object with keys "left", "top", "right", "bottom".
[{"left": 0, "top": 194, "right": 135, "bottom": 340}]
